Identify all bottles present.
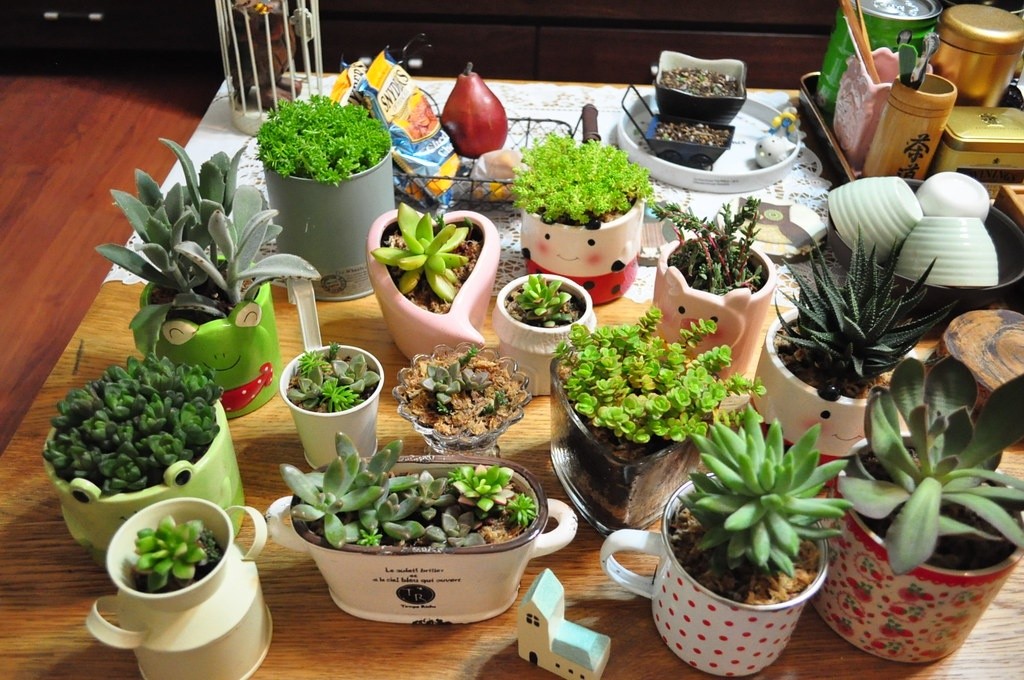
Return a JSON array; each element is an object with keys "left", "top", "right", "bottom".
[{"left": 932, "top": 4, "right": 1024, "bottom": 108}]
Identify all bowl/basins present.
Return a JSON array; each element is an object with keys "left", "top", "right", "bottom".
[{"left": 827, "top": 177, "right": 1024, "bottom": 325}]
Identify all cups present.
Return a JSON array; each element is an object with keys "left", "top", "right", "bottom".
[
  {"left": 916, "top": 171, "right": 990, "bottom": 226},
  {"left": 894, "top": 217, "right": 998, "bottom": 288},
  {"left": 828, "top": 176, "right": 923, "bottom": 264},
  {"left": 862, "top": 73, "right": 957, "bottom": 180},
  {"left": 833, "top": 47, "right": 933, "bottom": 171}
]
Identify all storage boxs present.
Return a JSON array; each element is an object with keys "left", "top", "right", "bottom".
[{"left": 934, "top": 103, "right": 1024, "bottom": 195}]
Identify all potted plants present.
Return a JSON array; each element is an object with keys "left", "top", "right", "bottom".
[
  {"left": 83, "top": 495, "right": 276, "bottom": 680},
  {"left": 391, "top": 340, "right": 537, "bottom": 455},
  {"left": 93, "top": 132, "right": 321, "bottom": 422},
  {"left": 262, "top": 429, "right": 580, "bottom": 632},
  {"left": 596, "top": 403, "right": 855, "bottom": 674},
  {"left": 508, "top": 130, "right": 653, "bottom": 306},
  {"left": 278, "top": 340, "right": 384, "bottom": 475},
  {"left": 747, "top": 218, "right": 968, "bottom": 462},
  {"left": 649, "top": 190, "right": 781, "bottom": 381},
  {"left": 652, "top": 48, "right": 749, "bottom": 124},
  {"left": 258, "top": 92, "right": 397, "bottom": 306},
  {"left": 362, "top": 200, "right": 503, "bottom": 370},
  {"left": 815, "top": 357, "right": 1024, "bottom": 663},
  {"left": 36, "top": 351, "right": 247, "bottom": 574},
  {"left": 490, "top": 267, "right": 599, "bottom": 398},
  {"left": 546, "top": 307, "right": 769, "bottom": 535}
]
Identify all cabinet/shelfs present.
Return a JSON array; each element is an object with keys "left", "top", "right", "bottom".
[{"left": 319, "top": 0, "right": 841, "bottom": 91}]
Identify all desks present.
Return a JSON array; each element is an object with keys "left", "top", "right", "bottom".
[{"left": 0, "top": 72, "right": 1024, "bottom": 680}]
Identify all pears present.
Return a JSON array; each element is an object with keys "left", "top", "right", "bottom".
[{"left": 441, "top": 62, "right": 508, "bottom": 159}]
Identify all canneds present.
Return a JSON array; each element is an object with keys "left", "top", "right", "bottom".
[{"left": 815, "top": 0, "right": 943, "bottom": 127}]
